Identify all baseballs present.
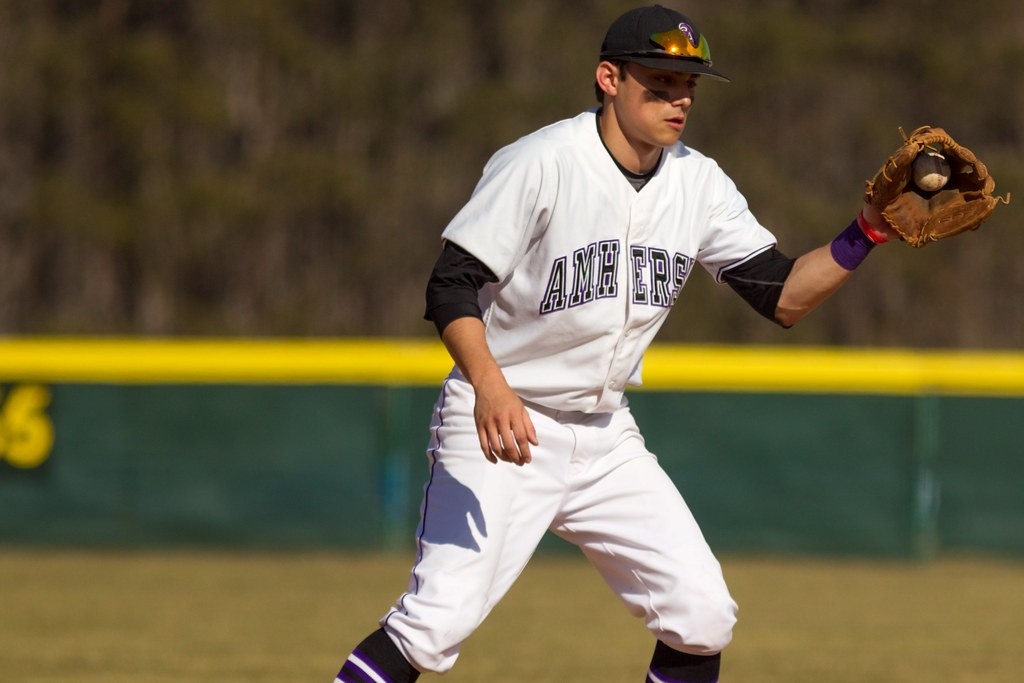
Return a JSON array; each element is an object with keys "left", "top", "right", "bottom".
[{"left": 913, "top": 149, "right": 952, "bottom": 191}]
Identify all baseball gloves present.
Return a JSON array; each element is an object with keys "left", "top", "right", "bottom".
[{"left": 864, "top": 126, "right": 1000, "bottom": 247}]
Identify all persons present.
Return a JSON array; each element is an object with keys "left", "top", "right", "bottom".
[{"left": 334, "top": 7, "right": 997, "bottom": 683}]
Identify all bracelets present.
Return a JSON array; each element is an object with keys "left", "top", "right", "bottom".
[
  {"left": 858, "top": 210, "right": 889, "bottom": 244},
  {"left": 830, "top": 219, "right": 876, "bottom": 271}
]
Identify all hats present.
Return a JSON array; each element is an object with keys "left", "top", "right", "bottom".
[{"left": 601, "top": 4, "right": 731, "bottom": 86}]
